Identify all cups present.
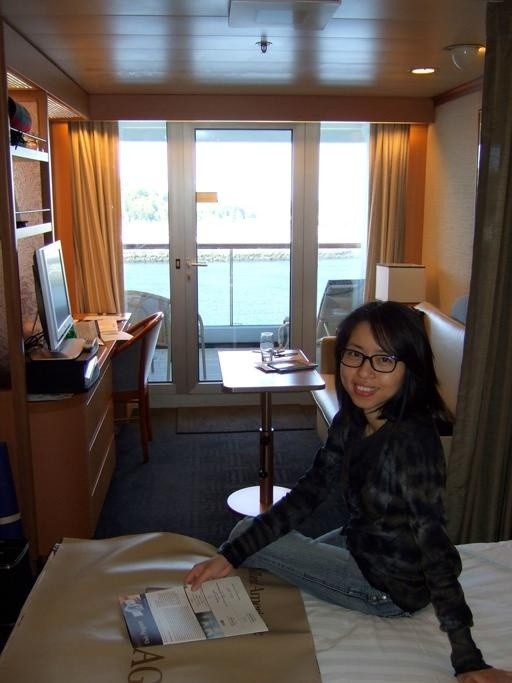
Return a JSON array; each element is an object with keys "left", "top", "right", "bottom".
[{"left": 260, "top": 331, "right": 277, "bottom": 357}]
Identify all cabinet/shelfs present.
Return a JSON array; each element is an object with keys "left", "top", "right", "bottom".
[{"left": 0, "top": 89, "right": 55, "bottom": 240}]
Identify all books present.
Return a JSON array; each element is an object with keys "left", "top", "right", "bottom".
[
  {"left": 255, "top": 354, "right": 318, "bottom": 373},
  {"left": 118, "top": 576, "right": 269, "bottom": 650}
]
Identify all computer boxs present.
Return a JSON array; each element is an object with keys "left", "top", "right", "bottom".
[{"left": 26, "top": 343, "right": 100, "bottom": 392}]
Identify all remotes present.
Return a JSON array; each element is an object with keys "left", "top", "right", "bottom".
[{"left": 82, "top": 336, "right": 97, "bottom": 350}]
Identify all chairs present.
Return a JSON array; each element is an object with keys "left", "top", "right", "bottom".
[
  {"left": 277, "top": 280, "right": 365, "bottom": 350},
  {"left": 110, "top": 311, "right": 165, "bottom": 463},
  {"left": 125, "top": 290, "right": 207, "bottom": 382}
]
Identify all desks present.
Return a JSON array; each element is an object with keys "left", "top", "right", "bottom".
[
  {"left": 0, "top": 312, "right": 133, "bottom": 563},
  {"left": 217, "top": 349, "right": 325, "bottom": 518}
]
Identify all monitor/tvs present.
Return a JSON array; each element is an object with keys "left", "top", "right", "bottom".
[{"left": 30, "top": 240, "right": 86, "bottom": 360}]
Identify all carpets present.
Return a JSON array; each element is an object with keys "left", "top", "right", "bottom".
[{"left": 176, "top": 403, "right": 315, "bottom": 434}]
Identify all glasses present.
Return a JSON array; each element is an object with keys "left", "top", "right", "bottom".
[{"left": 340, "top": 348, "right": 401, "bottom": 372}]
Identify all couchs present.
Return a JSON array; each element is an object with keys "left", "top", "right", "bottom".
[{"left": 310, "top": 301, "right": 466, "bottom": 467}]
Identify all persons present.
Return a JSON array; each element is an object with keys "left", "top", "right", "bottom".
[{"left": 182, "top": 302, "right": 512, "bottom": 682}]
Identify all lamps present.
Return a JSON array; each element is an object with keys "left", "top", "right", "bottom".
[{"left": 448, "top": 44, "right": 484, "bottom": 72}]
